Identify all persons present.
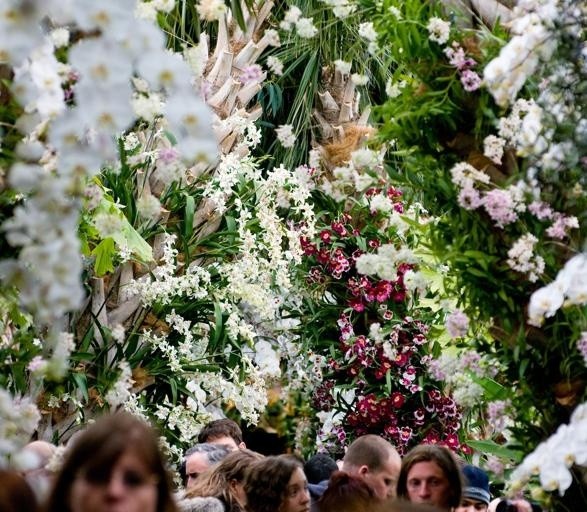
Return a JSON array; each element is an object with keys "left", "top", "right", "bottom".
[{"left": 1, "top": 409, "right": 490, "bottom": 511}]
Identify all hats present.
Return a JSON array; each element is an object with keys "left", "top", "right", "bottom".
[
  {"left": 306, "top": 480, "right": 328, "bottom": 496},
  {"left": 460, "top": 463, "right": 490, "bottom": 506}
]
